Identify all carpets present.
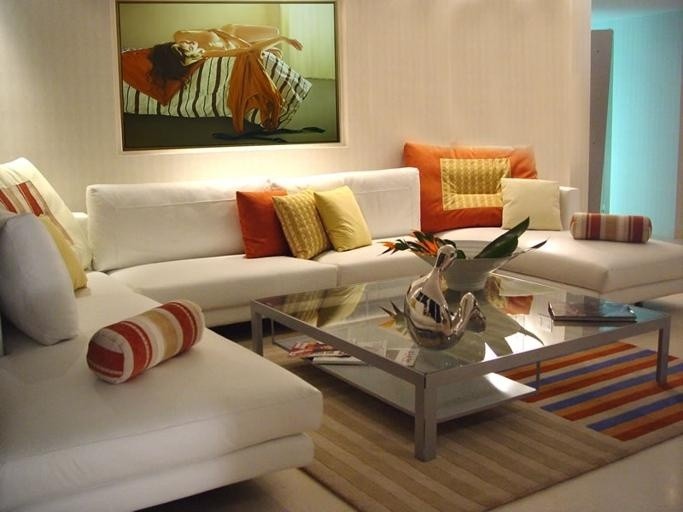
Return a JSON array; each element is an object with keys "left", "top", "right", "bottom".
[{"left": 280, "top": 337, "right": 682, "bottom": 512}]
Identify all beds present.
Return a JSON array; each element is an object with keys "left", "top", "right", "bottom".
[{"left": 119, "top": 44, "right": 310, "bottom": 149}]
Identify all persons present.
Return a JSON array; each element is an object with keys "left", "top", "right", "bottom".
[{"left": 148, "top": 23, "right": 304, "bottom": 76}]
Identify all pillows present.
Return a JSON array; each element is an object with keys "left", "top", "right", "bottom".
[
  {"left": 271, "top": 185, "right": 331, "bottom": 261},
  {"left": 314, "top": 186, "right": 372, "bottom": 253},
  {"left": 256, "top": 278, "right": 365, "bottom": 329},
  {"left": 236, "top": 184, "right": 289, "bottom": 258},
  {"left": 403, "top": 144, "right": 538, "bottom": 233},
  {"left": 569, "top": 213, "right": 655, "bottom": 243},
  {"left": 0, "top": 155, "right": 93, "bottom": 347},
  {"left": 85, "top": 298, "right": 206, "bottom": 385},
  {"left": 499, "top": 177, "right": 564, "bottom": 231}
]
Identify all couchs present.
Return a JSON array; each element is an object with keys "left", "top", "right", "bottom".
[{"left": 0, "top": 143, "right": 683, "bottom": 512}]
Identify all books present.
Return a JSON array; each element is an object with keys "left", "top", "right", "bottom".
[{"left": 548, "top": 300, "right": 637, "bottom": 324}]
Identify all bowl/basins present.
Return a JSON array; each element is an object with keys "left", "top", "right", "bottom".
[{"left": 417, "top": 254, "right": 518, "bottom": 292}]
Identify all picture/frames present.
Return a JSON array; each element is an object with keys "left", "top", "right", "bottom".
[{"left": 114, "top": 1, "right": 350, "bottom": 156}]
